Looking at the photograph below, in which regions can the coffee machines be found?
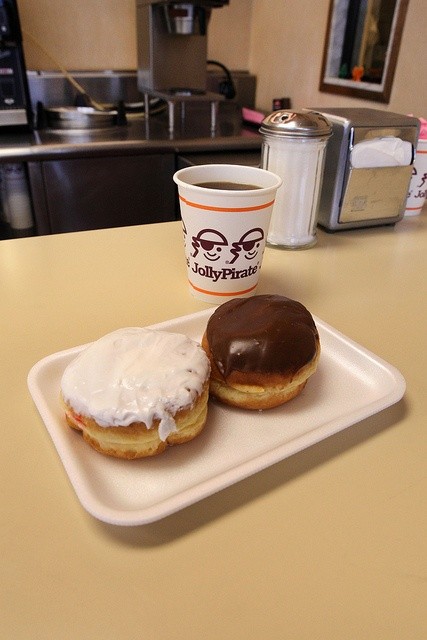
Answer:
[133,2,232,135]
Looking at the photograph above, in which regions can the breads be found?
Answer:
[59,327,210,458]
[202,295,321,411]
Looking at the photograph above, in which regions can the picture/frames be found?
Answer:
[319,1,410,105]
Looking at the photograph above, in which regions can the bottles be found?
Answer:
[3,159,35,229]
[259,109,330,249]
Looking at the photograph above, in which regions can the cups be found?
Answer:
[403,122,427,217]
[173,164,285,305]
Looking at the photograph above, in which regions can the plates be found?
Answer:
[44,103,121,135]
[26,305,407,526]
